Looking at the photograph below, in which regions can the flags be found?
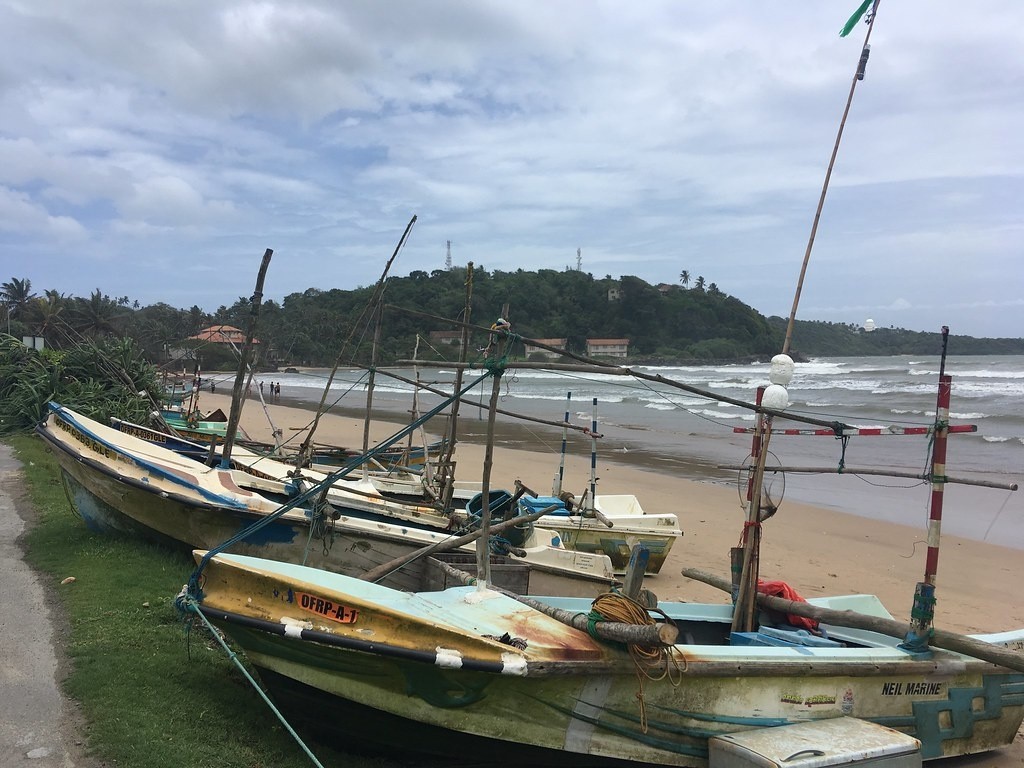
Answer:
[839,0,873,38]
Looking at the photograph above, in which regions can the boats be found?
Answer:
[177,72,1024,767]
[39,214,686,598]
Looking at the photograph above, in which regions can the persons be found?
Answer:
[193,378,215,393]
[275,382,280,398]
[269,381,275,396]
[260,381,264,395]
